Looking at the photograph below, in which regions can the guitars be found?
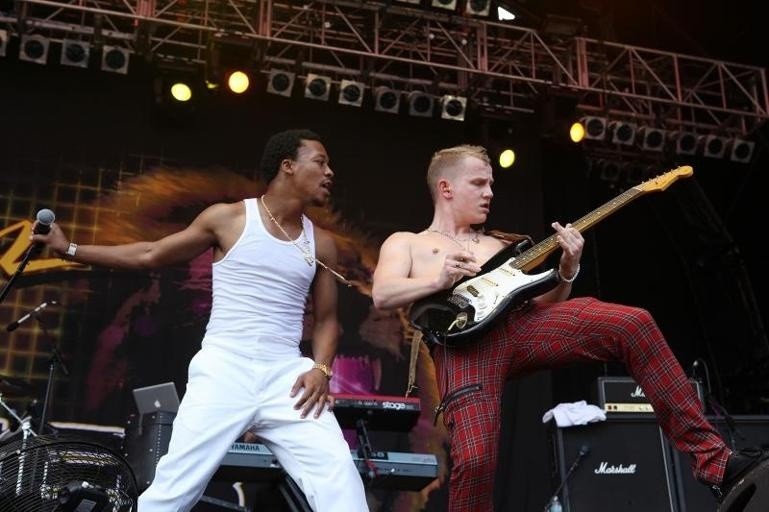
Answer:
[408,165,693,350]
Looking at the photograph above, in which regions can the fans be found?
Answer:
[0,433,139,512]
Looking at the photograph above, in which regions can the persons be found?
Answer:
[372,143,768,512]
[26,127,372,512]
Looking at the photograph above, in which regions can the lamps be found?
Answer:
[161,63,203,108]
[0,28,132,75]
[264,67,474,125]
[461,120,516,170]
[577,113,754,163]
[544,98,588,147]
[213,39,261,99]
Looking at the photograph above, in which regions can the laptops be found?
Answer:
[132,382,180,415]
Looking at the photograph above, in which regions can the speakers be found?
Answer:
[716,457,769,512]
[545,425,682,512]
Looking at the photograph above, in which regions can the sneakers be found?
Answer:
[696,445,768,487]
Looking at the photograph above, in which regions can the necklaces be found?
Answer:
[425,226,479,256]
[257,192,314,267]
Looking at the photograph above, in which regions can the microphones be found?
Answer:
[569,444,589,472]
[7,299,49,332]
[33,207,55,256]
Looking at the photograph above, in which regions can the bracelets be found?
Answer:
[65,242,76,256]
[312,363,332,379]
[558,264,580,283]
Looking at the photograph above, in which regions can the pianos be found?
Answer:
[210,442,437,485]
[329,393,421,433]
